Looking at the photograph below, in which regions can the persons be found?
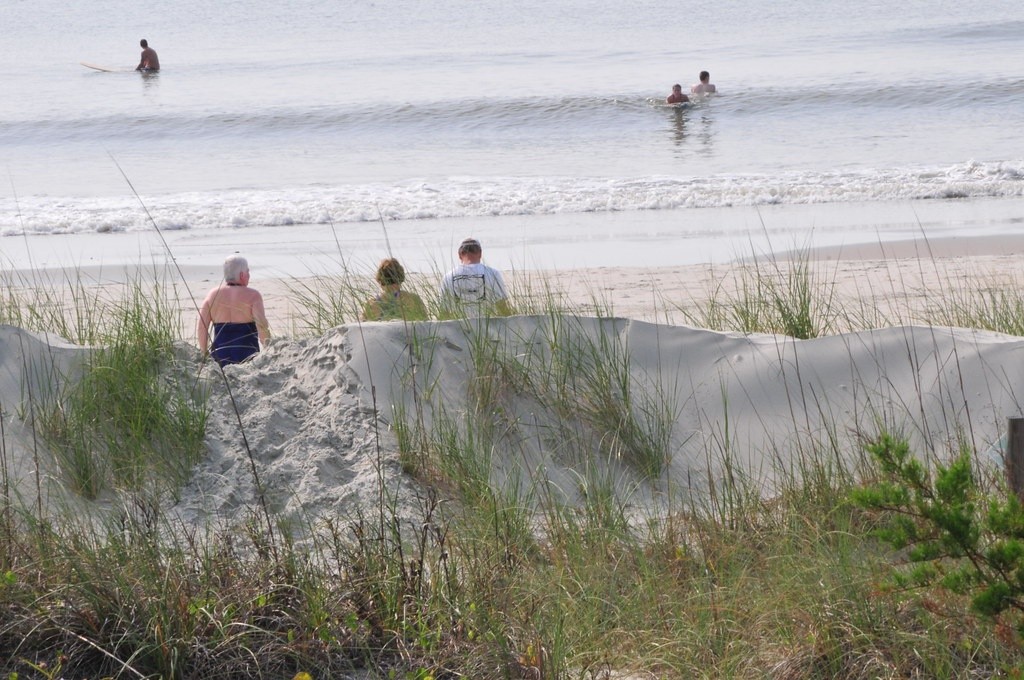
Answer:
[197,254,270,368]
[691,71,715,93]
[440,238,512,320]
[668,84,689,103]
[136,39,160,70]
[362,258,430,321]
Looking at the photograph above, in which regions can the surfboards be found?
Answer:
[79,60,136,72]
